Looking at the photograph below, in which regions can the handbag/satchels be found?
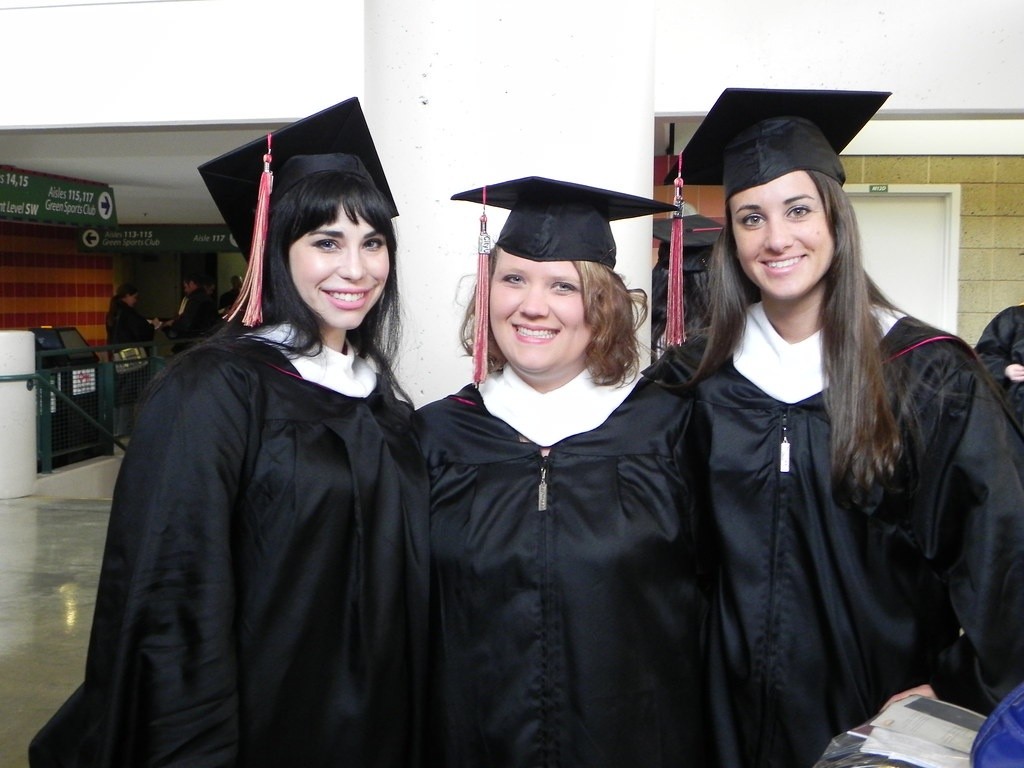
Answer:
[113,346,150,375]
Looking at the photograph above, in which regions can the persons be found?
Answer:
[161,270,243,354]
[412,174,710,768]
[974,303,1024,426]
[105,280,162,436]
[640,86,1024,768]
[652,214,725,362]
[29,95,412,768]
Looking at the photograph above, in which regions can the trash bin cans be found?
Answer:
[29,327,102,474]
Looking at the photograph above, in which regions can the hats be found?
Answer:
[650,214,723,271]
[198,97,397,328]
[452,177,679,382]
[663,88,891,348]
